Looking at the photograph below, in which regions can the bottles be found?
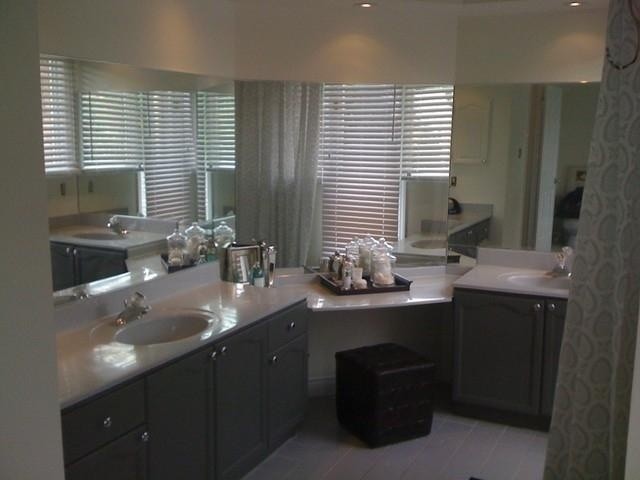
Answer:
[166,220,235,264]
[320,233,397,290]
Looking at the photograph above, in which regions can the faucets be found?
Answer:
[101,216,125,237]
[447,219,462,232]
[447,196,461,214]
[558,246,573,272]
[123,292,146,320]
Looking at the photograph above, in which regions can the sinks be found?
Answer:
[494,265,571,291]
[115,308,214,345]
[412,236,445,250]
[72,229,129,242]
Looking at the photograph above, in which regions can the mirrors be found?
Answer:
[445,81,600,268]
[39,54,282,336]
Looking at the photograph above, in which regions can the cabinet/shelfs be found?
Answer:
[265,298,310,458]
[49,240,168,292]
[450,286,568,432]
[143,321,268,480]
[60,378,149,480]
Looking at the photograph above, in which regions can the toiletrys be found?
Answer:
[165,222,188,264]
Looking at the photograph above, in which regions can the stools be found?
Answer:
[333,341,435,449]
